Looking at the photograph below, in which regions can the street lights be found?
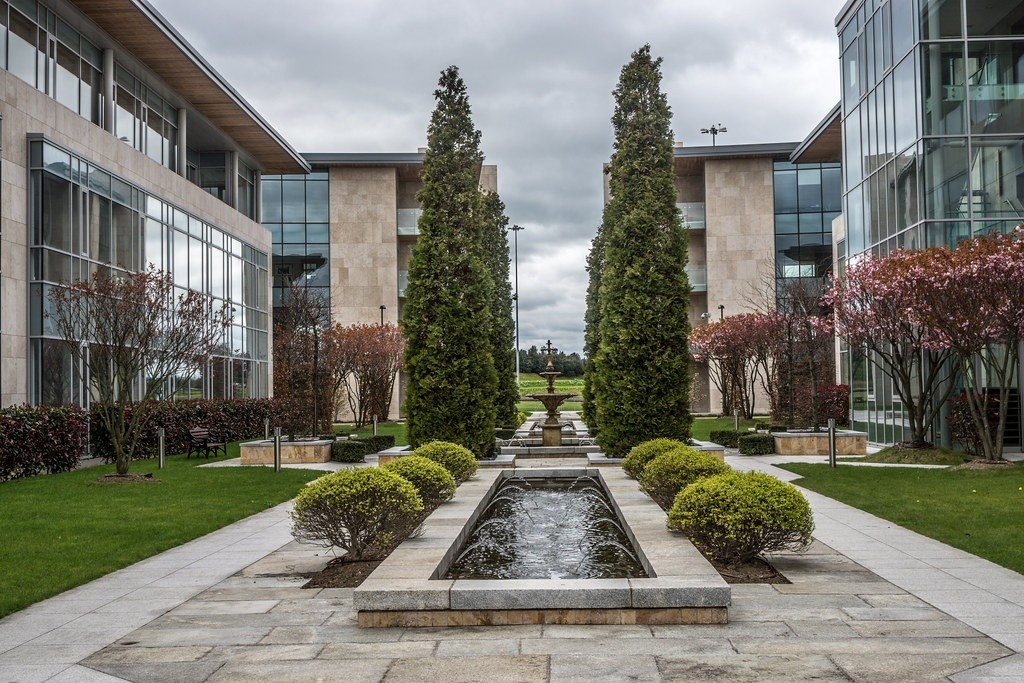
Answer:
[718,304,725,320]
[509,224,525,383]
[379,304,386,327]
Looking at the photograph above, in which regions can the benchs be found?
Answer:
[187,426,227,459]
